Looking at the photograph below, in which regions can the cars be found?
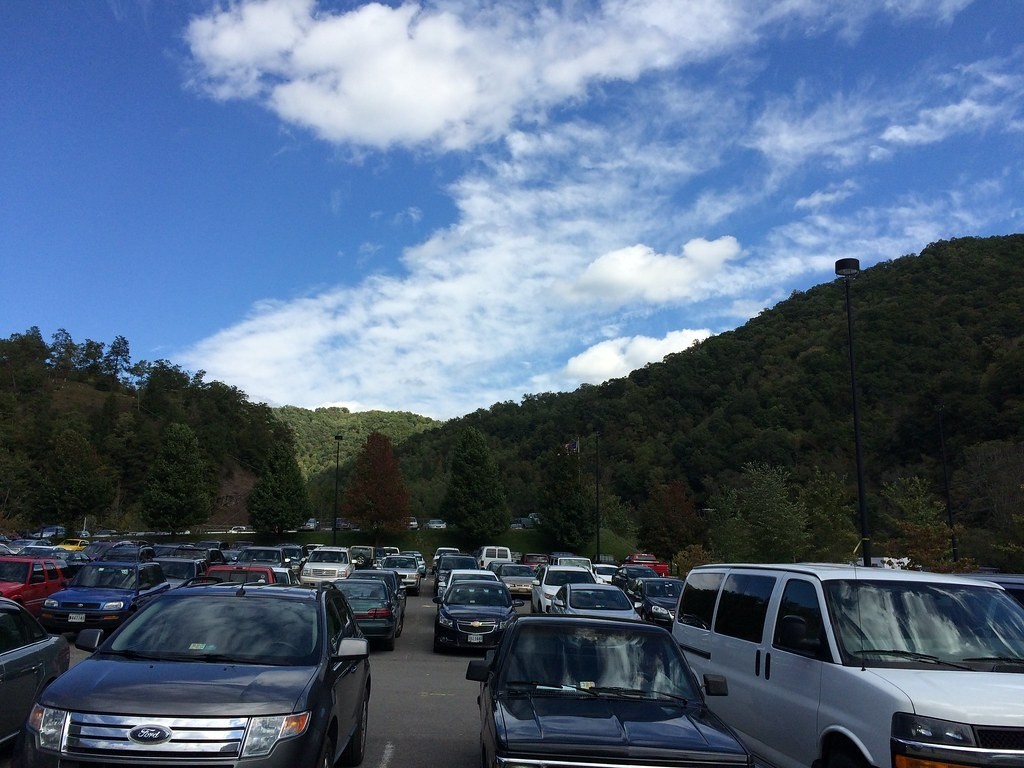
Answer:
[1,524,679,657]
[466,614,753,768]
[0,597,70,760]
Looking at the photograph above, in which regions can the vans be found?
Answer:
[670,561,1023,768]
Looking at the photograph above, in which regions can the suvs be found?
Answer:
[25,584,372,768]
